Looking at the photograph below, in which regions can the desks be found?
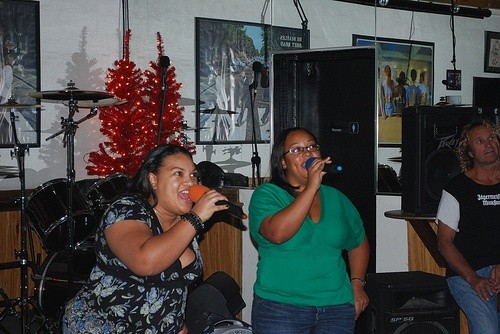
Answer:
[383,208,460,334]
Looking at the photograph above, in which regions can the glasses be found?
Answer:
[284,143,320,156]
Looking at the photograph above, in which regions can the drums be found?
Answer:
[35,241,96,320]
[87,173,140,219]
[24,177,95,253]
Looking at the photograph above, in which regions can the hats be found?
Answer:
[396,71,408,84]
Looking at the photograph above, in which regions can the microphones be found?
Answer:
[253,62,261,97]
[305,158,342,172]
[159,55,170,92]
[188,185,247,220]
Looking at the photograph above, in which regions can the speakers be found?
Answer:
[353,271,461,334]
[402,105,482,216]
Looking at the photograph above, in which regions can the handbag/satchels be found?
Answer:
[378,164,401,193]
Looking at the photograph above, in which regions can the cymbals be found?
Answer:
[0,102,46,109]
[28,90,128,108]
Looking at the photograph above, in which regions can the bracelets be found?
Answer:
[350,278,366,287]
[184,211,204,233]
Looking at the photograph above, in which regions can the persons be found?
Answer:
[417,72,429,105]
[378,65,397,119]
[406,69,421,106]
[435,119,500,334]
[63,143,229,334]
[393,71,406,113]
[248,127,369,334]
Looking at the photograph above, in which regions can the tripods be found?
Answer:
[0,109,55,334]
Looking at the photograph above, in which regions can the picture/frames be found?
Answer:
[484,30,500,73]
[195,17,270,144]
[0,0,41,148]
[352,34,435,147]
[447,69,462,90]
